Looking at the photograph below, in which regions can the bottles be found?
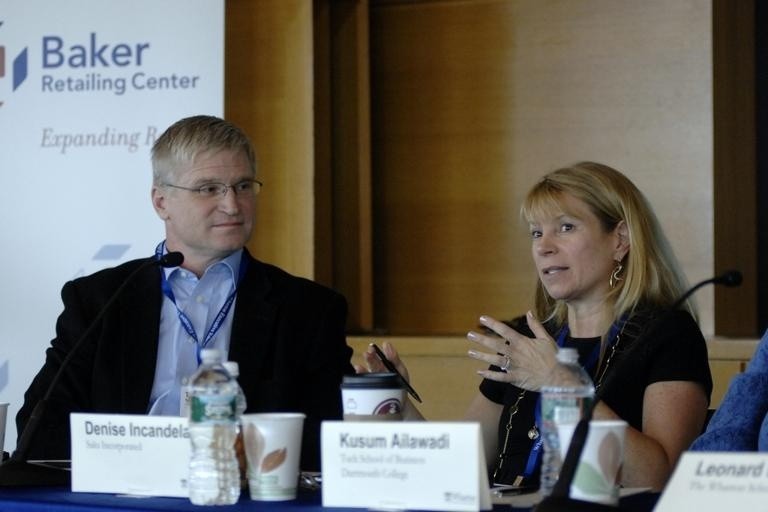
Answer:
[539,344,596,500]
[183,348,248,507]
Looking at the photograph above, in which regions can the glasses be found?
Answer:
[163,180,265,199]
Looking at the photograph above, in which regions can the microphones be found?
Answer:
[532,269,744,512]
[0,251,184,491]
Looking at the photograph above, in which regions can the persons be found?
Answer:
[354,162,713,494]
[11,115,348,471]
[689,328,768,451]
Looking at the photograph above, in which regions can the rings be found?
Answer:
[504,339,510,355]
[501,355,510,371]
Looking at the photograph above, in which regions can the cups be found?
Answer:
[0,402,12,464]
[556,417,628,506]
[340,372,406,417]
[237,413,306,503]
